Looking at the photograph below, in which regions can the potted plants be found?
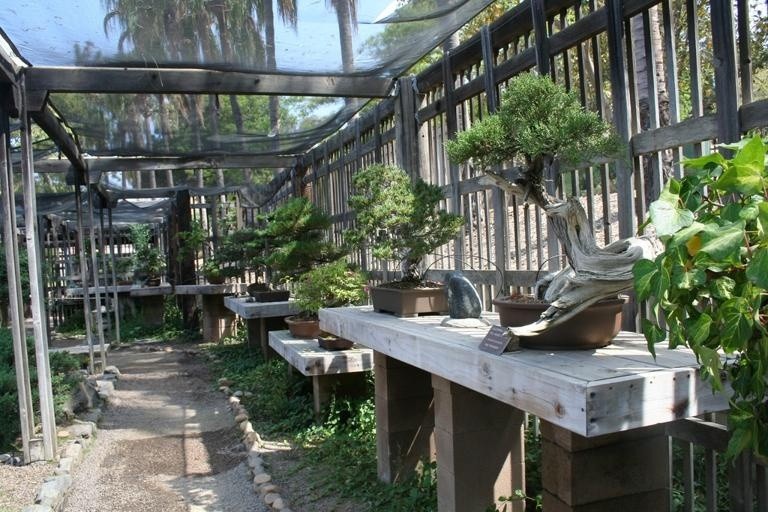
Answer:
[443,71,664,350]
[279,257,369,351]
[223,214,290,303]
[342,162,467,318]
[633,132,768,512]
[173,212,250,284]
[128,224,167,287]
[266,195,353,340]
